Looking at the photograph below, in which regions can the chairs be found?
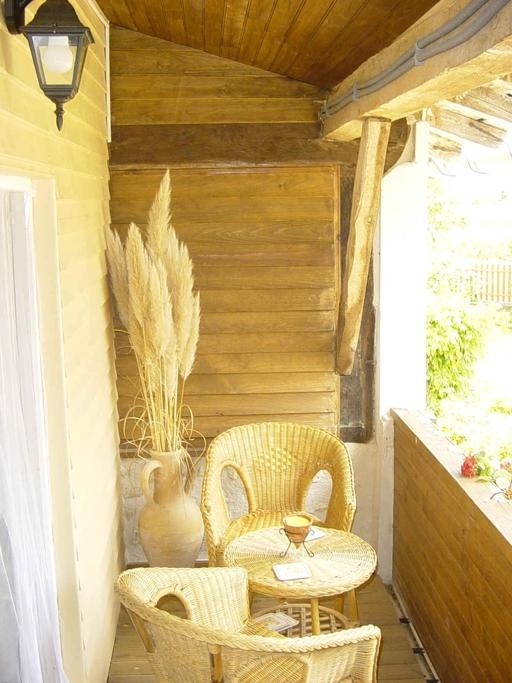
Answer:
[115,566,382,683]
[200,421,357,568]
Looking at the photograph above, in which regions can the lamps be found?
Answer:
[3,0,95,132]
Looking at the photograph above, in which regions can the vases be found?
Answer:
[137,450,206,567]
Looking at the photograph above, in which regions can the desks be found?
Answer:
[222,525,378,636]
